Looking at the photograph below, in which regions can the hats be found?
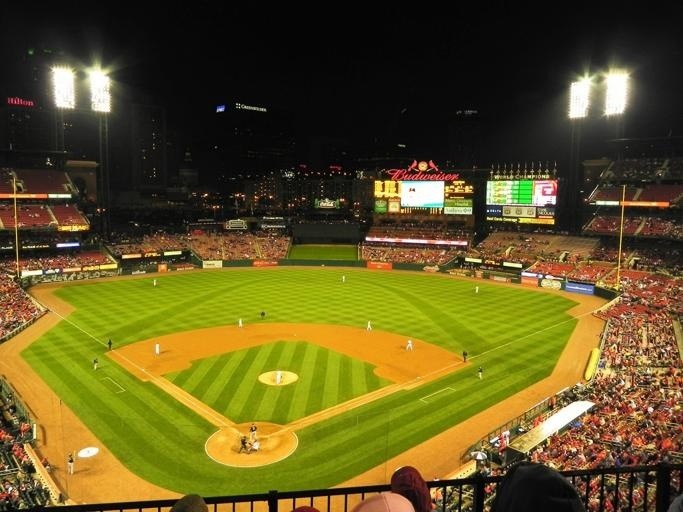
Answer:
[491,463,588,512]
[391,466,432,512]
[349,492,416,512]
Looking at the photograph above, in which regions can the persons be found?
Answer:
[238,317,243,329]
[406,338,413,349]
[106,338,112,352]
[291,506,319,512]
[0,273,77,510]
[92,357,99,371]
[477,366,482,379]
[260,310,265,322]
[275,368,282,384]
[351,465,432,511]
[461,350,468,363]
[356,214,681,296]
[239,424,259,454]
[155,341,159,357]
[365,318,372,331]
[0,202,293,278]
[152,277,157,287]
[426,412,683,511]
[341,273,345,283]
[549,295,682,412]
